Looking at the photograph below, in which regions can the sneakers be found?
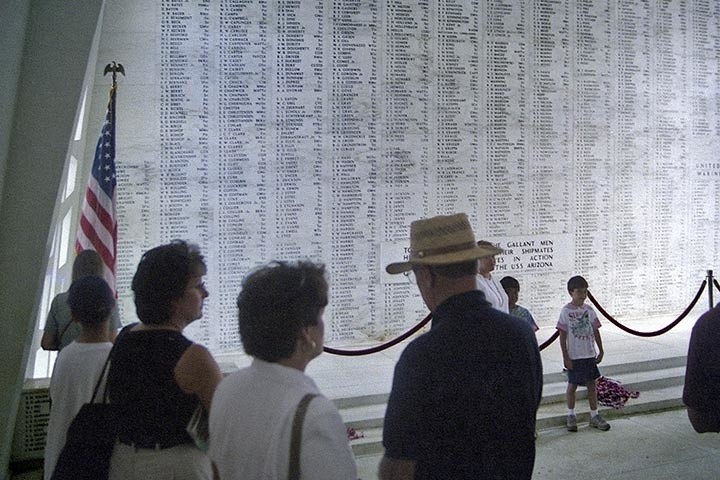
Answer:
[567,415,578,432]
[589,415,610,430]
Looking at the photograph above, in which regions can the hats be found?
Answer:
[387,213,503,275]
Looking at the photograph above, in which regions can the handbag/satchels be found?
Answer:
[50,322,139,480]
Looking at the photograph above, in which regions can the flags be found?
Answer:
[75,82,118,300]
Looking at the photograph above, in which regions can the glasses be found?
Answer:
[404,269,422,284]
[186,282,206,291]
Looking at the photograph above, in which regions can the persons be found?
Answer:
[682,303,720,433]
[475,240,509,314]
[378,213,544,480]
[501,276,539,332]
[556,275,610,432]
[40,250,121,350]
[45,276,114,480]
[206,260,357,480]
[109,242,219,480]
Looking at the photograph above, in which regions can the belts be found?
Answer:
[120,439,192,449]
